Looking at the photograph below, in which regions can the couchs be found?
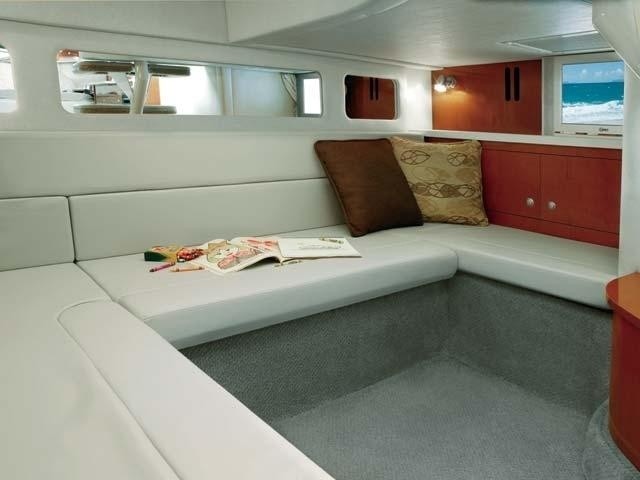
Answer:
[375,130,619,416]
[1,132,333,480]
[68,134,454,421]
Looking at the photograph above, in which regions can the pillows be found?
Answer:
[313,139,425,238]
[391,138,488,226]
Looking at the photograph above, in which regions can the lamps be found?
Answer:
[435,74,458,92]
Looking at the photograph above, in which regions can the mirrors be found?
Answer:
[343,71,400,122]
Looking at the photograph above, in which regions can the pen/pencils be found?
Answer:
[148,261,205,272]
[274,260,302,267]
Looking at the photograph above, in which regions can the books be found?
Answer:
[182,235,363,276]
